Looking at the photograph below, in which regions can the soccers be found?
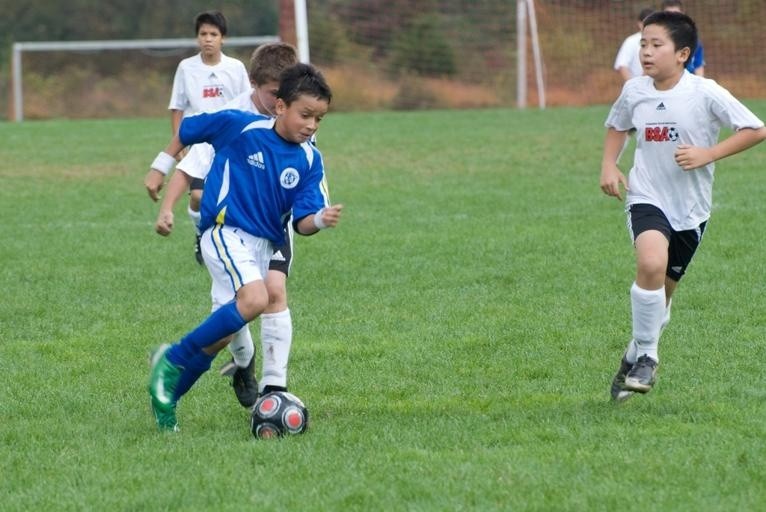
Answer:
[251,391,308,440]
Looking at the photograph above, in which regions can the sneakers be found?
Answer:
[151,397,180,435]
[148,341,186,410]
[625,353,658,392]
[222,350,260,407]
[611,351,636,402]
[194,234,204,267]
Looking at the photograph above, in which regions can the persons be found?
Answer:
[659,0,706,76]
[615,7,654,82]
[167,12,254,267]
[597,10,765,408]
[152,44,315,413]
[141,61,345,437]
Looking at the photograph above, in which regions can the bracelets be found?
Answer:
[313,206,329,232]
[149,150,177,176]
